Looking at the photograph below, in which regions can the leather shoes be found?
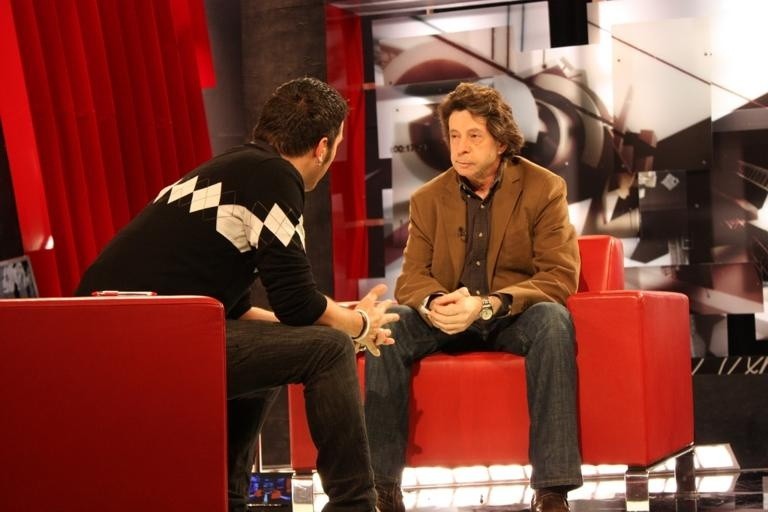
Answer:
[531,487,571,512]
[373,481,406,512]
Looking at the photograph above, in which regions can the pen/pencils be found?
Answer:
[91,290,157,296]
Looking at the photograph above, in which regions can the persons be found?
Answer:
[363,77,590,511]
[71,76,402,512]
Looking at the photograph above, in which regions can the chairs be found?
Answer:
[286,235,697,511]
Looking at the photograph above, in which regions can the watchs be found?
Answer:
[477,293,495,323]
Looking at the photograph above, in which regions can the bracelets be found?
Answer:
[355,306,371,345]
